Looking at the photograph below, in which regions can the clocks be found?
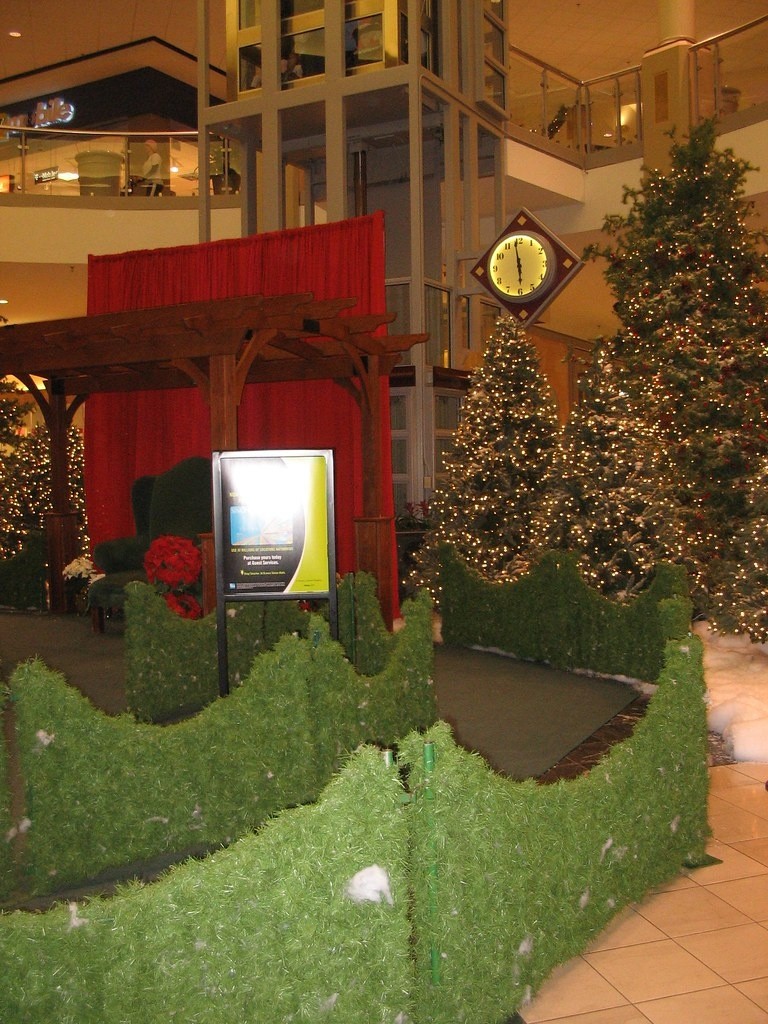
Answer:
[468,206,585,328]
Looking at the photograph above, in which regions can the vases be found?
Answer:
[76,599,86,612]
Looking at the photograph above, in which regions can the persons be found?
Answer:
[142,140,163,196]
[281,48,303,81]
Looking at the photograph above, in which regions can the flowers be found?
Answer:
[61,557,98,598]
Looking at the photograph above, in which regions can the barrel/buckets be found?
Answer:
[75,150,123,197]
